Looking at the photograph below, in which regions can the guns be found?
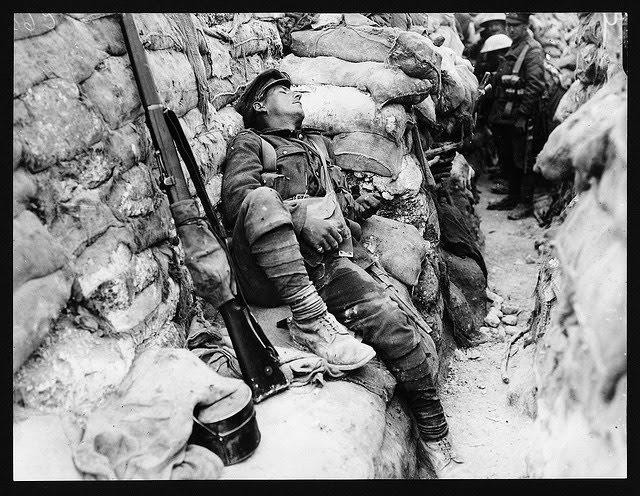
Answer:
[118,11,289,403]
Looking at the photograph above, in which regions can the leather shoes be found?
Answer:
[507,203,534,219]
[491,182,512,194]
[416,436,491,481]
[290,314,377,370]
[489,196,518,209]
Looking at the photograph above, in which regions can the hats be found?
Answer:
[505,11,530,25]
[233,69,291,111]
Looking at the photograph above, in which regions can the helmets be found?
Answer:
[479,14,506,26]
[481,34,513,53]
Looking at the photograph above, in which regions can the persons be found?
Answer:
[464,11,547,222]
[219,68,469,479]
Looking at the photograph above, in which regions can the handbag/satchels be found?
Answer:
[282,139,351,240]
